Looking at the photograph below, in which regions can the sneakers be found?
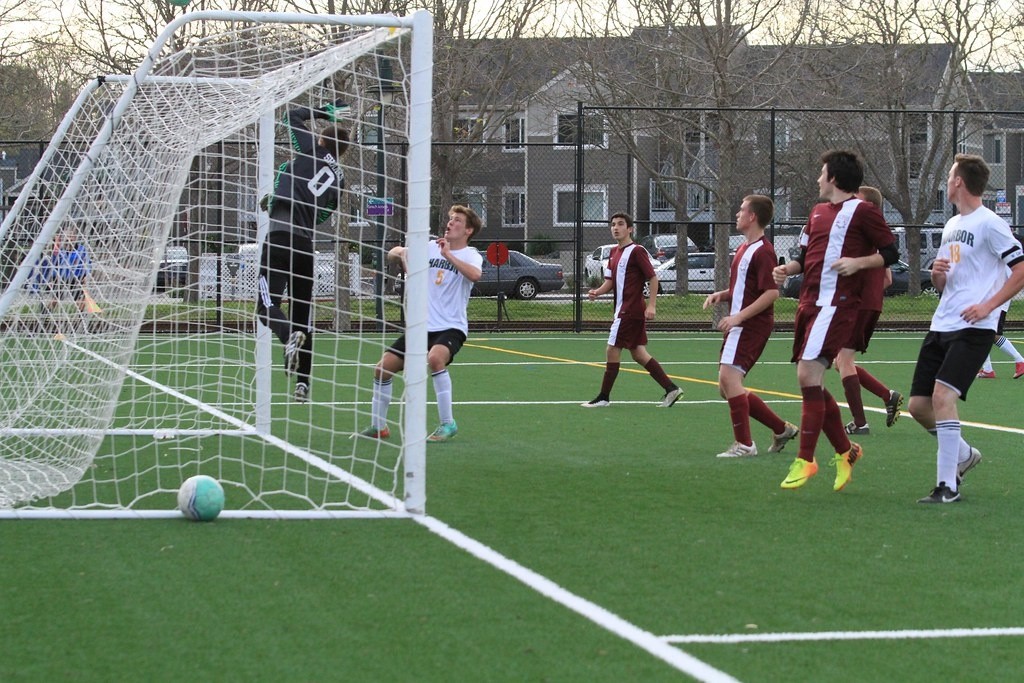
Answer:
[284,331,306,377]
[780,456,818,489]
[580,395,610,407]
[360,423,390,438]
[976,369,995,378]
[656,387,684,408]
[956,446,983,486]
[828,441,863,491]
[844,421,869,434]
[1012,360,1024,378]
[916,481,961,502]
[293,382,309,402]
[884,390,904,427]
[768,421,799,452]
[427,420,457,443]
[716,440,757,457]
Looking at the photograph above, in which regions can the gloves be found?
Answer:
[321,97,351,122]
[259,194,268,212]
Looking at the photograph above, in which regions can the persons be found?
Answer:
[975,264,1024,379]
[256,97,353,401]
[833,186,903,435]
[580,212,684,408]
[907,152,1024,504]
[703,195,799,457]
[772,148,904,492]
[358,204,484,444]
[28,221,90,317]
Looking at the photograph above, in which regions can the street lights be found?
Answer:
[364,49,403,329]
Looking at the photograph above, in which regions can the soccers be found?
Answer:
[178,474,226,522]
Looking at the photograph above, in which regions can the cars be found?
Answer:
[153,245,188,286]
[782,260,941,299]
[638,233,699,264]
[392,250,564,299]
[889,228,943,270]
[585,244,661,283]
[642,252,736,299]
